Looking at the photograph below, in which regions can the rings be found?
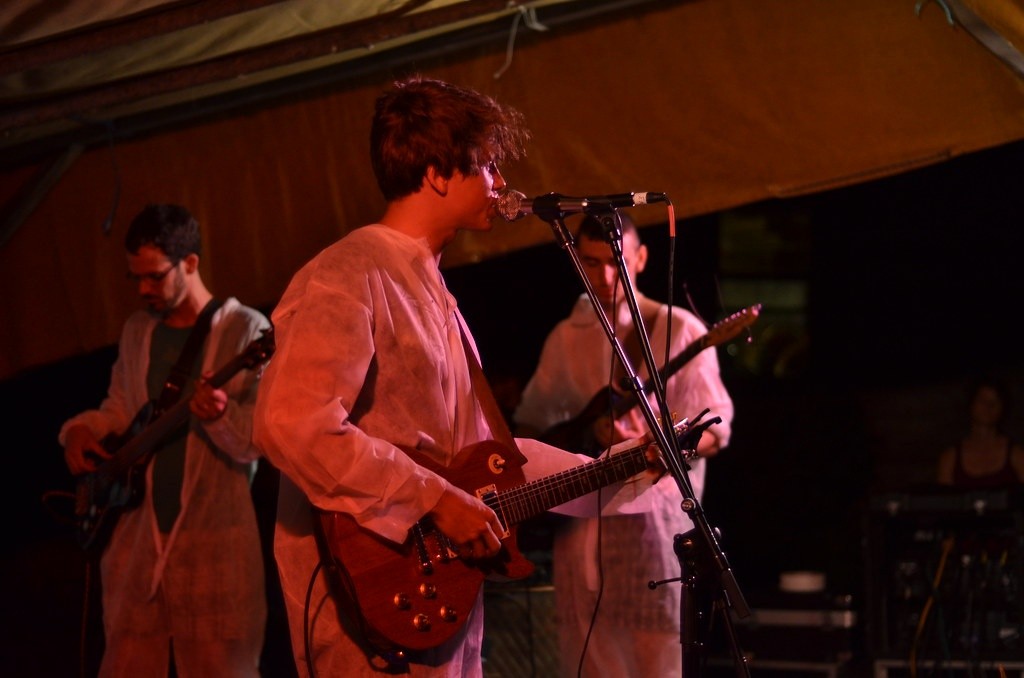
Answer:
[200,402,209,410]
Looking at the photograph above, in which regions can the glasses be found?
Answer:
[126,258,180,282]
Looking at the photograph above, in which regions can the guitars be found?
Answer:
[316,406,723,666]
[538,301,764,462]
[32,327,275,570]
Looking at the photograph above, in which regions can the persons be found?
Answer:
[252,69,668,678]
[934,378,1024,563]
[511,208,735,677]
[58,199,276,678]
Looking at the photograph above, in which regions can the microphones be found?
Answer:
[495,189,667,223]
